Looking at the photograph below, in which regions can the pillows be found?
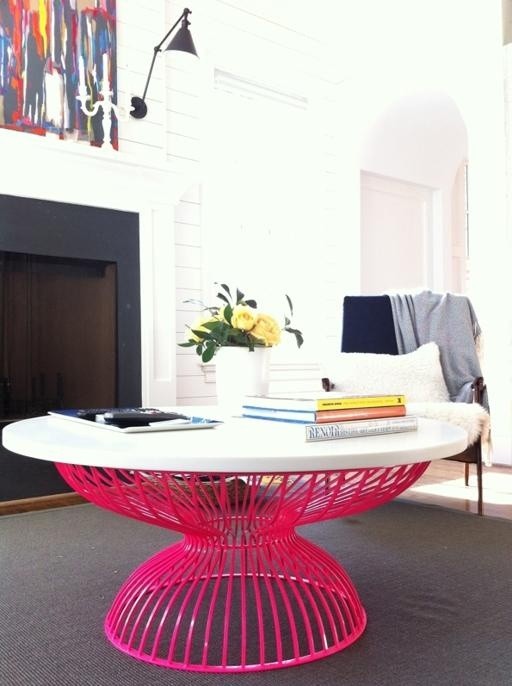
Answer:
[337,341,451,404]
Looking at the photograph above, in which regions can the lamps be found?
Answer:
[130,6,202,121]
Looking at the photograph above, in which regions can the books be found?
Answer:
[230,391,418,445]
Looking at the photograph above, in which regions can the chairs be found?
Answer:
[340,294,493,517]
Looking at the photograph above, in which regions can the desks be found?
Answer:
[2,405,469,676]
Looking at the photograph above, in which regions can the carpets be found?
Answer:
[0,480,511,686]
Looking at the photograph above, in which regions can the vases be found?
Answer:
[214,346,256,418]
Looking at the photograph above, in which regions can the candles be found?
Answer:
[78,52,109,89]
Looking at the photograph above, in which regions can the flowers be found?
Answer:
[178,277,304,365]
